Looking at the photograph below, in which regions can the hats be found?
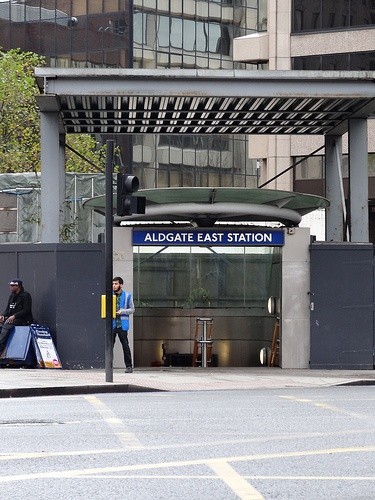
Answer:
[9,279,23,286]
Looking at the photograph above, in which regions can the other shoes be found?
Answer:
[125,367,133,373]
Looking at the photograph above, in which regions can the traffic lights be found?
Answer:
[117,172,146,217]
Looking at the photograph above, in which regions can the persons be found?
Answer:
[112,277,135,373]
[0,280,33,352]
